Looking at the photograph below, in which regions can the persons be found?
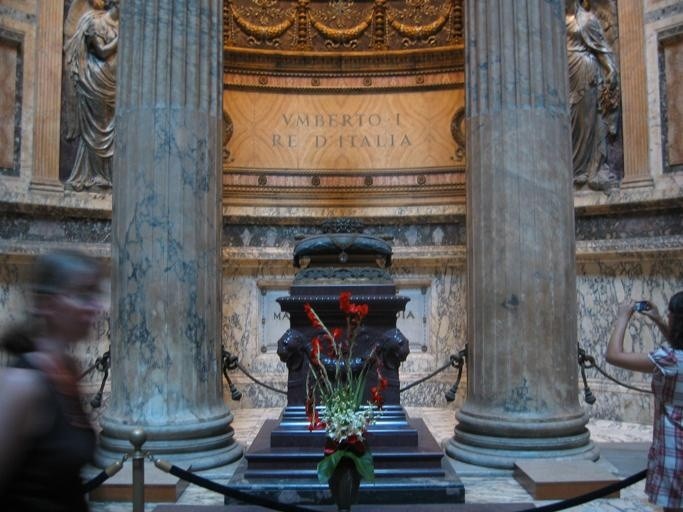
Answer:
[65,3,119,192]
[0,250,111,512]
[566,0,619,187]
[605,289,683,511]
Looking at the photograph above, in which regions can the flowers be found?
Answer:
[303,291,388,487]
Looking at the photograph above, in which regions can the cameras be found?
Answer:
[633,300,649,312]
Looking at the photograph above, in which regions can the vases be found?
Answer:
[328,456,360,512]
